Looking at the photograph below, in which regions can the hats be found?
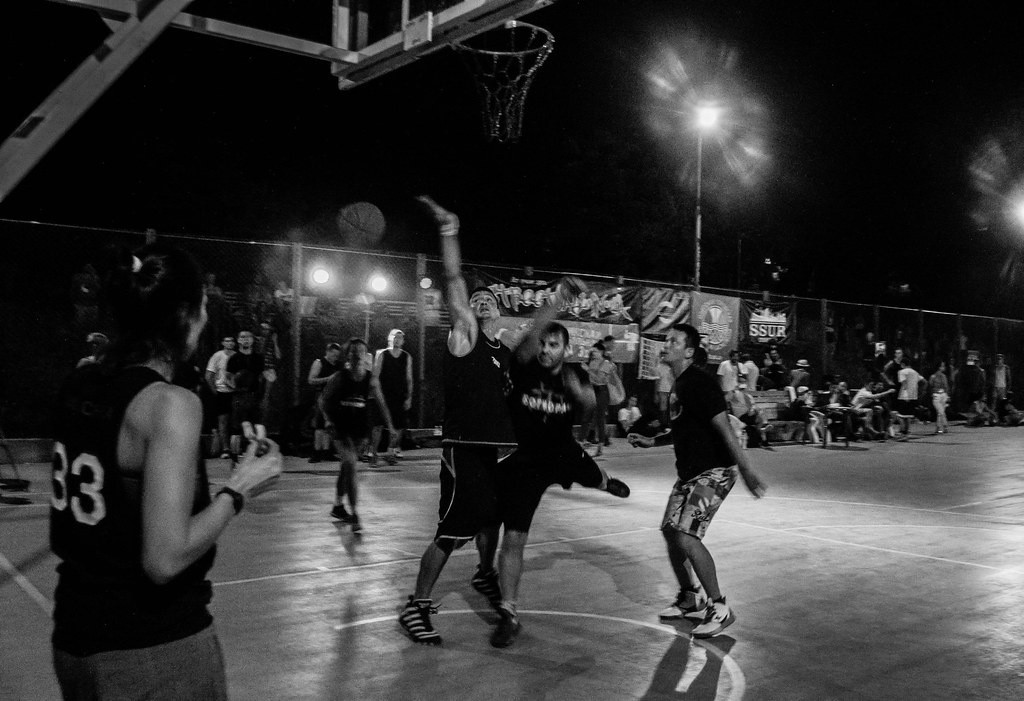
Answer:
[796,359,810,367]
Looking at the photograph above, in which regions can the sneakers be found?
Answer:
[605,478,630,498]
[399,595,442,647]
[661,586,706,620]
[690,597,735,639]
[491,617,521,648]
[471,563,502,610]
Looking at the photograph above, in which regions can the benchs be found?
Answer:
[749,388,865,442]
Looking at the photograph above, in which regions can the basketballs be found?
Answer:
[339,202,386,248]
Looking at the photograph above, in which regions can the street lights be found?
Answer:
[689,99,721,294]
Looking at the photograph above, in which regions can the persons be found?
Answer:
[375,329,412,466]
[717,348,1013,445]
[74,328,111,367]
[617,394,669,438]
[318,337,398,532]
[580,335,624,450]
[308,344,342,463]
[993,353,1011,427]
[51,241,284,700]
[627,324,764,638]
[225,330,268,455]
[488,276,629,647]
[204,338,236,460]
[898,359,927,435]
[929,359,951,433]
[399,199,524,648]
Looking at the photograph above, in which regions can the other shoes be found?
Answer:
[352,514,361,532]
[220,449,230,458]
[331,505,352,521]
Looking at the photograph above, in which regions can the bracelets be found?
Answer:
[216,487,244,516]
[441,224,459,238]
